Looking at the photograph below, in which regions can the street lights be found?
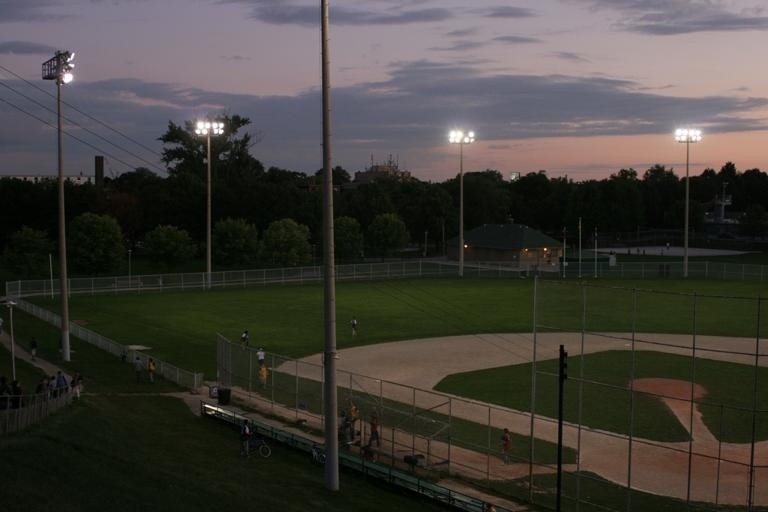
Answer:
[2,300,17,382]
[41,50,75,364]
[675,127,702,279]
[447,129,476,280]
[194,120,225,291]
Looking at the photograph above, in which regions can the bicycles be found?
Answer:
[312,446,327,466]
[248,439,271,458]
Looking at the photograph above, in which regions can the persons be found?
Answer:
[346,401,360,440]
[257,364,270,391]
[368,406,381,447]
[239,418,250,458]
[350,316,357,336]
[241,330,249,350]
[133,357,145,385]
[257,345,265,365]
[146,358,157,383]
[500,428,512,464]
[29,337,38,361]
[1,369,86,409]
